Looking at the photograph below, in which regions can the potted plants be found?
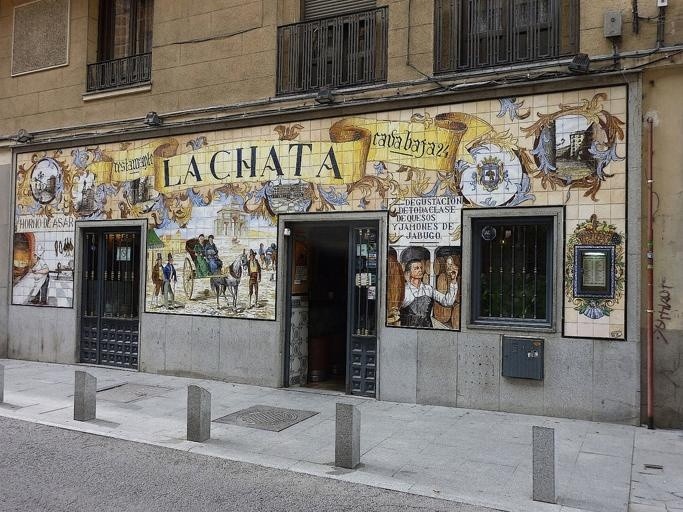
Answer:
[308,309,334,382]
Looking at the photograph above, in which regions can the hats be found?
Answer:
[249,249,257,256]
[33,244,45,259]
[156,253,162,261]
[168,252,173,261]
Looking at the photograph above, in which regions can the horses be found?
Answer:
[209,248,250,309]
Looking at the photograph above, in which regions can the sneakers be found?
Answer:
[31,298,46,305]
[154,303,182,312]
[247,304,261,309]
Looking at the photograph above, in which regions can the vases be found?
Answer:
[329,335,346,376]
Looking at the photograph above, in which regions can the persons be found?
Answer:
[398,257,458,328]
[29,252,50,305]
[150,233,278,310]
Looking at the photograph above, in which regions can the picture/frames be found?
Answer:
[11,0,70,78]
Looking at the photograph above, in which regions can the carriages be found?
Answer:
[182,238,248,309]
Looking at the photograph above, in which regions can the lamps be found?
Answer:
[284,222,291,237]
[567,53,590,74]
[145,112,162,127]
[15,129,35,142]
[315,85,334,104]
